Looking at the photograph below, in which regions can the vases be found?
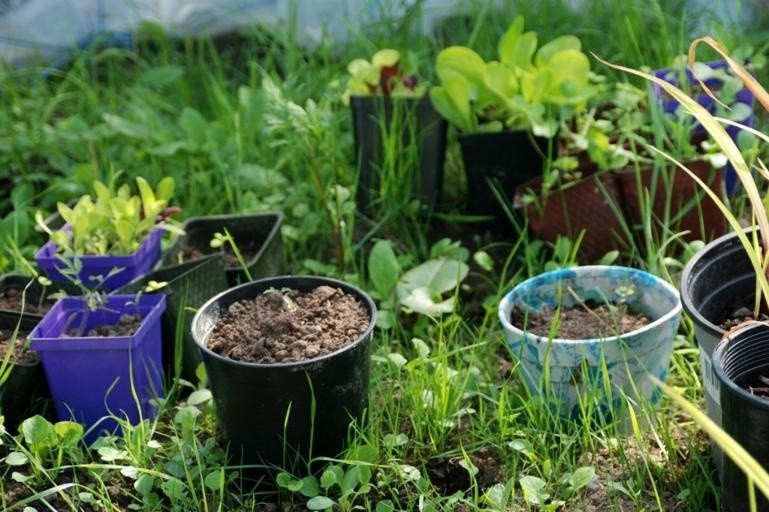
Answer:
[187,273,380,463]
[711,323,769,511]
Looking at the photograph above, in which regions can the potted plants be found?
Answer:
[35,172,178,297]
[0,273,58,312]
[596,34,769,426]
[0,312,53,425]
[341,17,764,266]
[30,295,173,442]
[182,211,292,278]
[112,255,227,392]
[496,264,684,426]
